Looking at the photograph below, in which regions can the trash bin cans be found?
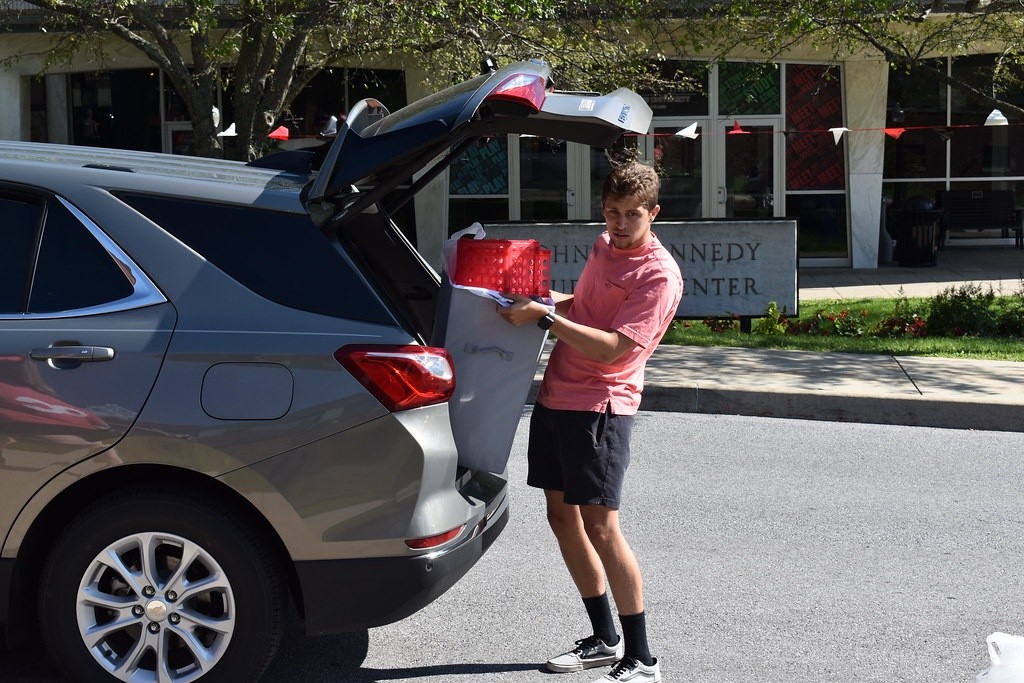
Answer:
[898,195,953,269]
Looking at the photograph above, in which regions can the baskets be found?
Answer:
[454,236,552,299]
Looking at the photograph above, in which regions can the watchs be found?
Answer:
[537,311,555,331]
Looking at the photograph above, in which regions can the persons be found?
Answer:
[496,160,683,683]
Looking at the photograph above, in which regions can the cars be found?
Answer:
[487,149,701,220]
[727,159,897,226]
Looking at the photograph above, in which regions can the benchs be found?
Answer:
[935,188,1024,252]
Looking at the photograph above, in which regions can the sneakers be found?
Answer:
[588,656,663,683]
[547,634,623,672]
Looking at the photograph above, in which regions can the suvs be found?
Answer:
[0,61,657,683]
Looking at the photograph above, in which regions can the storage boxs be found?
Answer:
[429,238,556,474]
